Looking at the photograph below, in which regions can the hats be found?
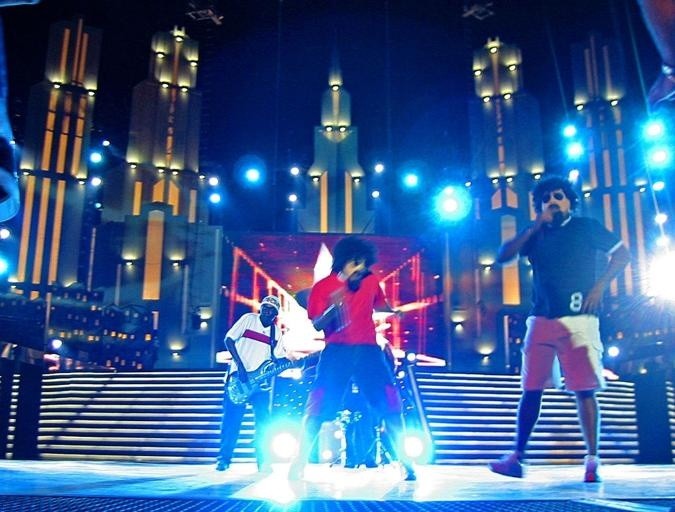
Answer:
[260,295,280,316]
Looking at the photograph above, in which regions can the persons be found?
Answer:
[286,237,421,482]
[210,294,282,472]
[487,174,633,485]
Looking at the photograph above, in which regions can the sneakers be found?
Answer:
[287,462,305,481]
[215,460,230,472]
[488,453,524,479]
[258,464,276,473]
[400,463,417,481]
[583,457,603,483]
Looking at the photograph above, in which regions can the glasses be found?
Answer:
[542,192,564,204]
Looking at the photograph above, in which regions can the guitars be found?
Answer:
[225,358,305,406]
